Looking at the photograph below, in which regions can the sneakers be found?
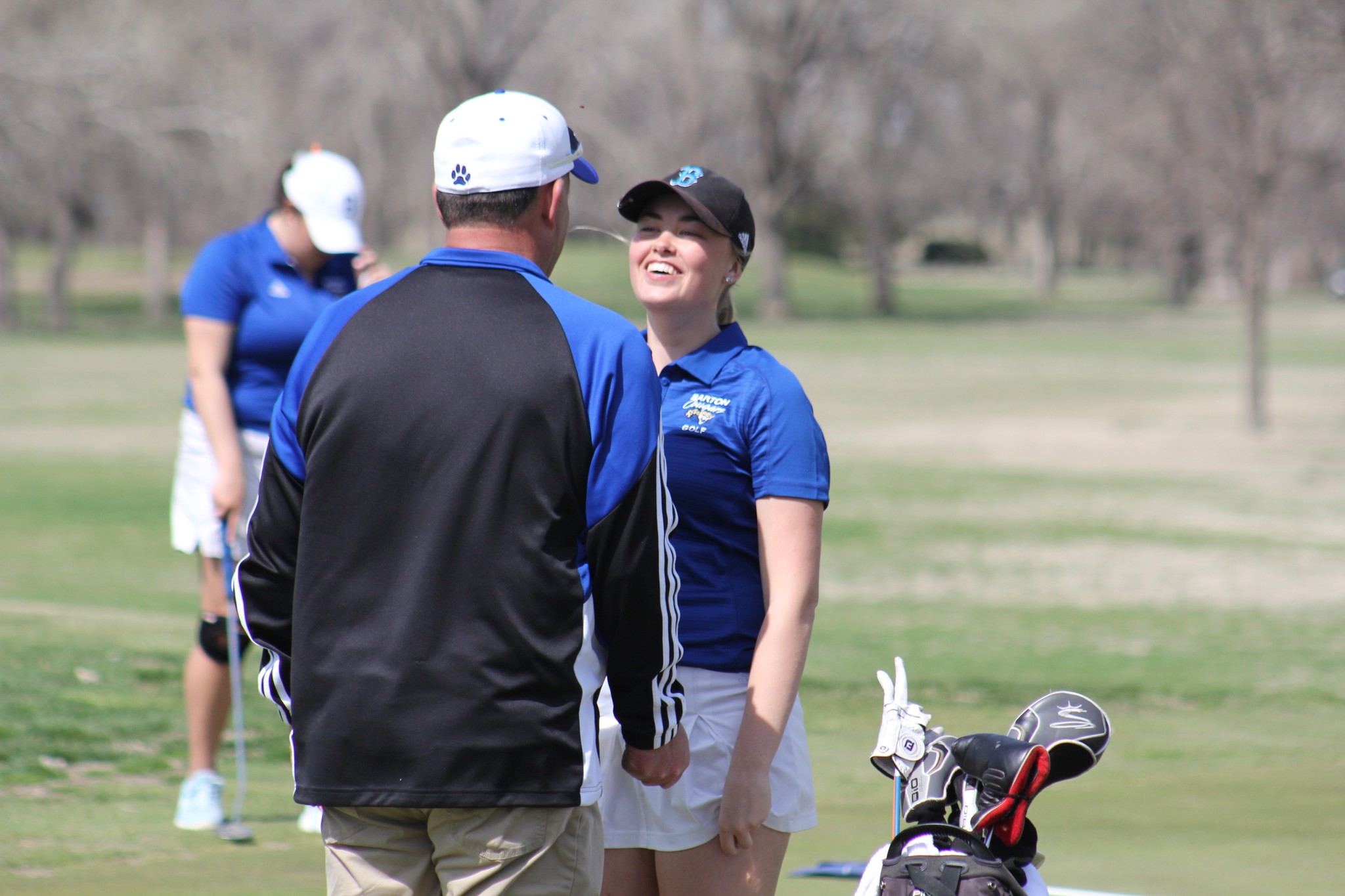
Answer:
[173,770,229,830]
[297,805,323,833]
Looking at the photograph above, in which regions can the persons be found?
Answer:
[567,164,832,896]
[228,88,693,895]
[167,146,387,838]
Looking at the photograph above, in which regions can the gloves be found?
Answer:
[870,657,944,782]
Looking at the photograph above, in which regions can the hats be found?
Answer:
[282,151,365,254]
[618,165,755,270]
[433,90,599,195]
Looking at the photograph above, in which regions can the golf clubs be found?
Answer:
[216,516,256,844]
